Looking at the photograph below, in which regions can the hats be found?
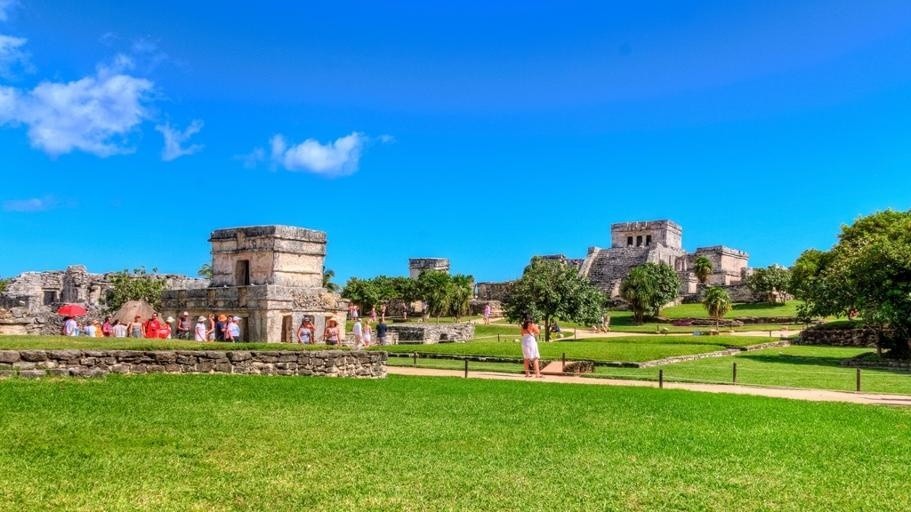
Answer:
[135,311,242,322]
[326,318,339,325]
[304,317,310,322]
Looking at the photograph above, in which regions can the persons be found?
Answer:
[402,302,407,319]
[349,303,387,347]
[482,304,490,324]
[549,321,561,332]
[595,314,610,332]
[61,311,242,342]
[297,317,316,344]
[324,317,341,344]
[421,300,427,321]
[520,313,541,379]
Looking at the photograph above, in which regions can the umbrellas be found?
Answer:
[58,305,88,318]
